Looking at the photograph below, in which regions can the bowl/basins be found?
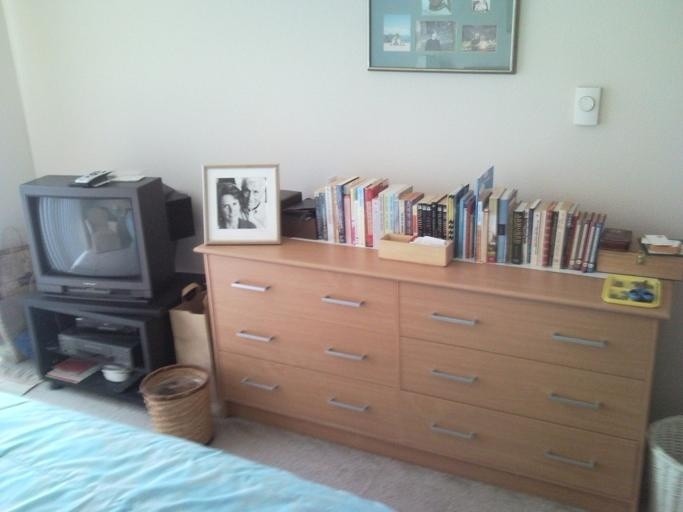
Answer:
[101,364,132,383]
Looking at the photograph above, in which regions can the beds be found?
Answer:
[0,392,395,512]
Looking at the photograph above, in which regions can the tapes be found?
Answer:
[101,364,132,382]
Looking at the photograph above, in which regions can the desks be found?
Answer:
[21,273,205,403]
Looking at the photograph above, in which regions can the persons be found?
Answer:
[423,29,440,50]
[216,182,255,230]
[238,178,266,230]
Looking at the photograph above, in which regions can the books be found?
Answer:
[641,236,681,255]
[312,172,607,273]
[600,226,633,251]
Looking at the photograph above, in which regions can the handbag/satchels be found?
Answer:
[169,283,226,423]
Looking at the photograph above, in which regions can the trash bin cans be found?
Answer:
[646,414,683,512]
[137,363,213,445]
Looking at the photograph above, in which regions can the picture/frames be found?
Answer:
[368,0,520,75]
[202,162,282,246]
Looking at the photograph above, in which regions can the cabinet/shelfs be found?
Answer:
[193,235,673,512]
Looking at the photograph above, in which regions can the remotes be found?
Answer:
[75,168,113,184]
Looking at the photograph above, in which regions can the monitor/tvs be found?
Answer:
[18,175,195,305]
